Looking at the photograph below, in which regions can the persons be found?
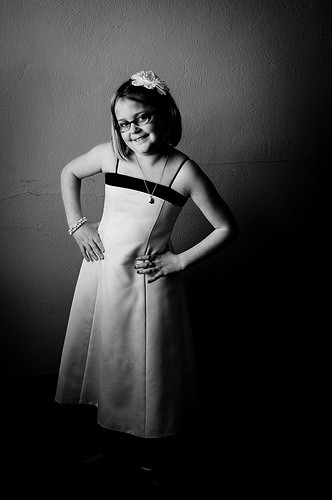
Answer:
[54,70,239,500]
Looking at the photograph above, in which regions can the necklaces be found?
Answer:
[133,149,171,203]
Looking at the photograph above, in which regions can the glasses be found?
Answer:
[113,111,153,132]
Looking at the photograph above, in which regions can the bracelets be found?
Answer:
[68,216,87,235]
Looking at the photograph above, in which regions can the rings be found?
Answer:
[148,261,152,267]
